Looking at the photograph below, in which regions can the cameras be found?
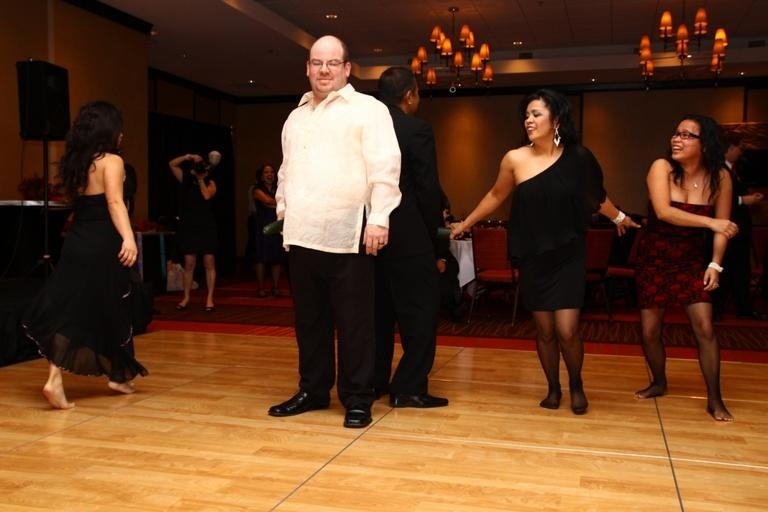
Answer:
[192,160,206,178]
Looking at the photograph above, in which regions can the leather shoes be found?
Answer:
[343,404,372,429]
[371,385,390,400]
[205,303,215,312]
[175,301,190,311]
[267,389,332,416]
[389,389,449,408]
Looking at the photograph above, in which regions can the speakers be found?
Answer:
[17,58,71,142]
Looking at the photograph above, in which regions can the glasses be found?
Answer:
[670,130,700,142]
[310,59,341,72]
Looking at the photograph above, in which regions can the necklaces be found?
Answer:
[679,168,705,188]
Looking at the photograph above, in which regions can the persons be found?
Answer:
[376,67,450,408]
[713,132,767,320]
[446,89,642,414]
[629,115,741,421]
[422,205,464,323]
[243,164,292,297]
[169,152,221,313]
[19,100,139,410]
[117,147,136,217]
[268,35,404,427]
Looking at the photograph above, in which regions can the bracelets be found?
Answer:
[707,261,724,272]
[198,179,203,183]
[612,210,626,225]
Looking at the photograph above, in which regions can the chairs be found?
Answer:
[587,229,636,321]
[467,221,521,328]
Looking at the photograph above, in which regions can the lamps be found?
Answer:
[410,7,494,88]
[639,0,728,92]
